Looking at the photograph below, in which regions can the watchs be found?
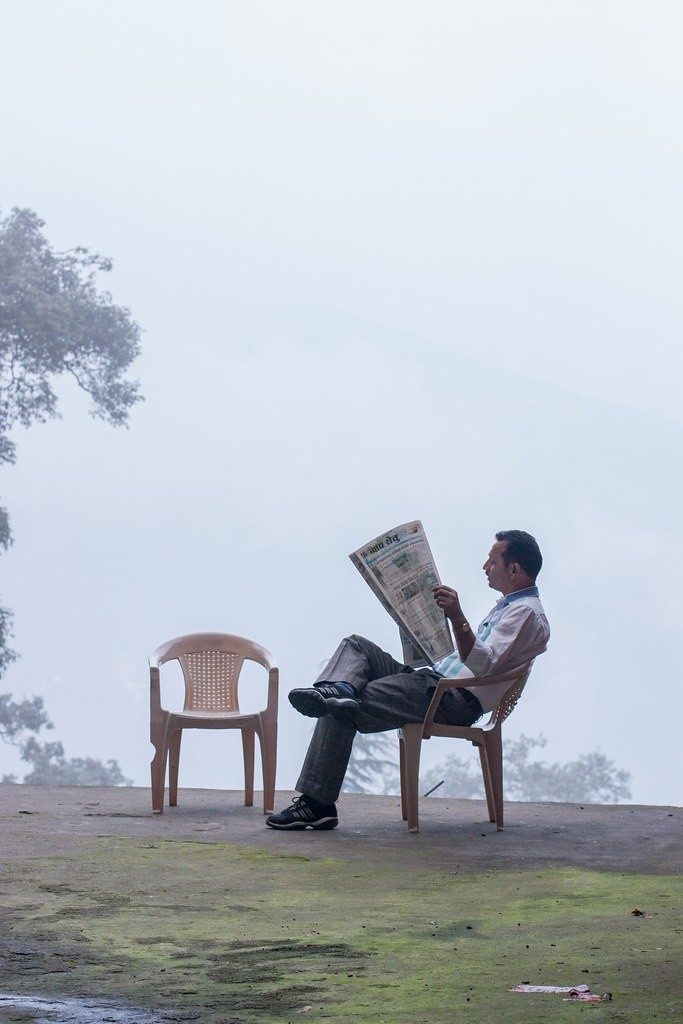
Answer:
[453,623,471,634]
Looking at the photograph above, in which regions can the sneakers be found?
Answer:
[266,794,338,830]
[288,683,359,717]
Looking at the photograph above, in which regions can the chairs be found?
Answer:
[148,633,280,814]
[396,658,535,831]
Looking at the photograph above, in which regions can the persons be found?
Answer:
[266,530,550,830]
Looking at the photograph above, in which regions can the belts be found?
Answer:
[455,687,481,719]
